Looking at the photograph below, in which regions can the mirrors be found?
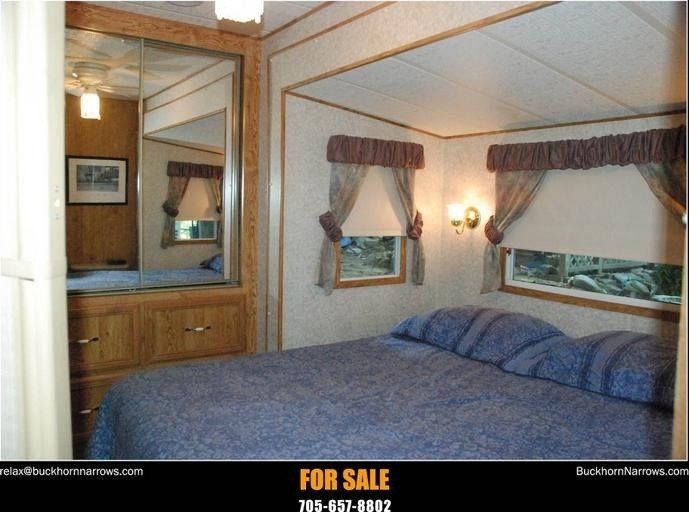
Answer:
[64,3,265,298]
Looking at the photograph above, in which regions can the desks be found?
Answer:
[69,258,132,274]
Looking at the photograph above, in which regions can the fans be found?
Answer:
[63,62,141,98]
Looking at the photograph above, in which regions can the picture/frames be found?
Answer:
[65,154,129,206]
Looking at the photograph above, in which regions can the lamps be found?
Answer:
[78,92,101,118]
[447,198,479,238]
[212,2,267,26]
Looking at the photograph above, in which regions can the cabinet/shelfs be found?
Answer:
[62,292,261,461]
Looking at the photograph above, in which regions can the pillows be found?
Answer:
[202,252,223,274]
[392,303,571,370]
[514,329,675,411]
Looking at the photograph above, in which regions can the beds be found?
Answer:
[68,251,223,292]
[90,301,681,464]
